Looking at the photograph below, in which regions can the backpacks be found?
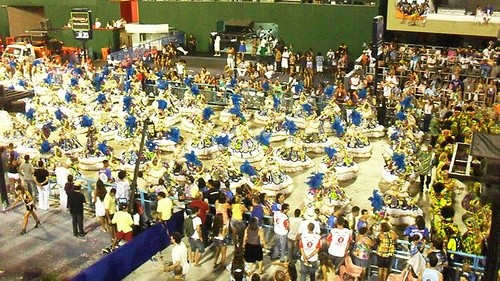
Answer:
[184,215,197,237]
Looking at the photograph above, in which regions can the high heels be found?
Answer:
[34,221,41,228]
[20,230,26,235]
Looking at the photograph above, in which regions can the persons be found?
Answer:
[0,0,499,281]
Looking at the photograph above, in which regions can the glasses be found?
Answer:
[284,208,290,210]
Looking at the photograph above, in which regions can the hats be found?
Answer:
[73,178,82,186]
